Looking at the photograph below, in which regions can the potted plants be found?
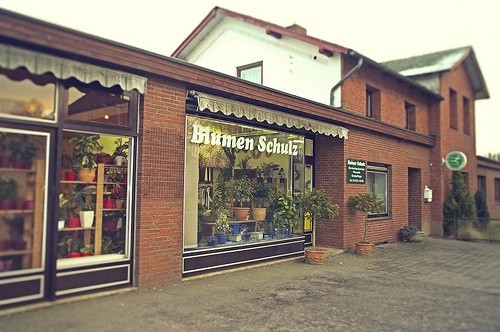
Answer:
[399,223,426,243]
[57,134,129,259]
[0,131,36,277]
[198,149,298,246]
[348,192,385,255]
[298,187,340,265]
